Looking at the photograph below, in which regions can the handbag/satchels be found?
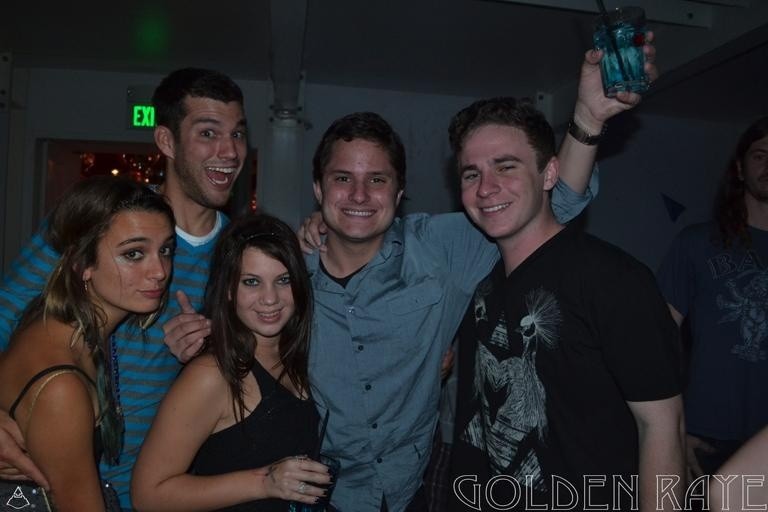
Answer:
[0,370,121,510]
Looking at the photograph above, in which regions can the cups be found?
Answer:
[290,457,340,512]
[595,27,648,97]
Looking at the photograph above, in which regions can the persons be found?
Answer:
[0,32,768,512]
